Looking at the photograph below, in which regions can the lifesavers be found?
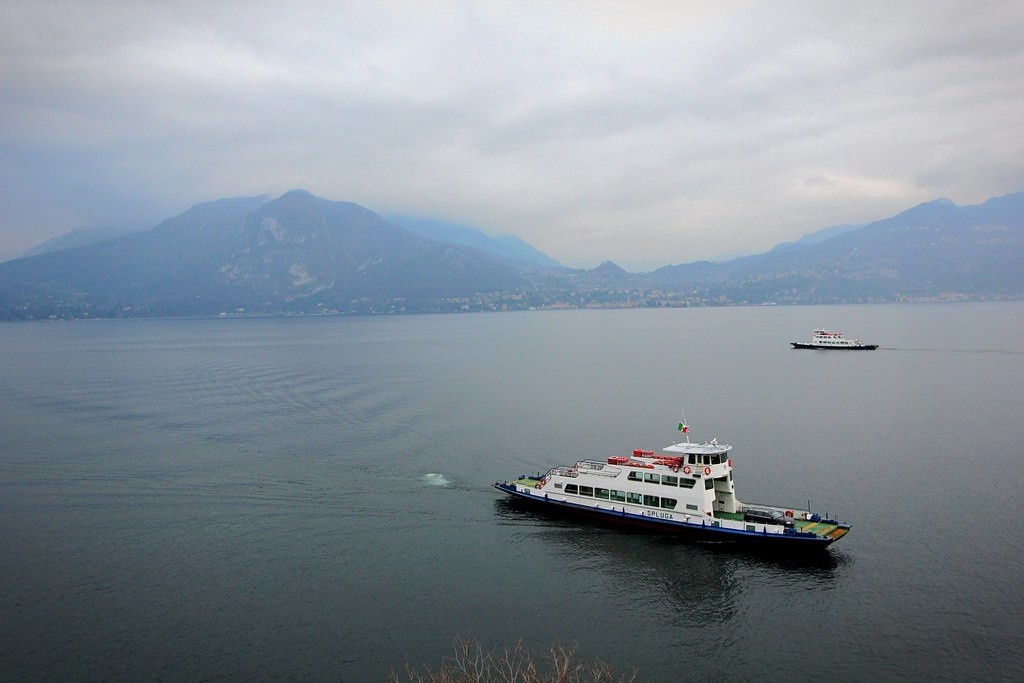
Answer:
[535,483,541,489]
[540,478,546,485]
[683,466,691,473]
[672,465,679,473]
[785,511,793,518]
[705,467,711,475]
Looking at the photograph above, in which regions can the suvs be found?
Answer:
[744,509,796,527]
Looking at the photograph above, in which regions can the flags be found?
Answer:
[677,421,688,433]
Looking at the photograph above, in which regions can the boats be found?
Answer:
[493,408,854,555]
[790,326,879,350]
